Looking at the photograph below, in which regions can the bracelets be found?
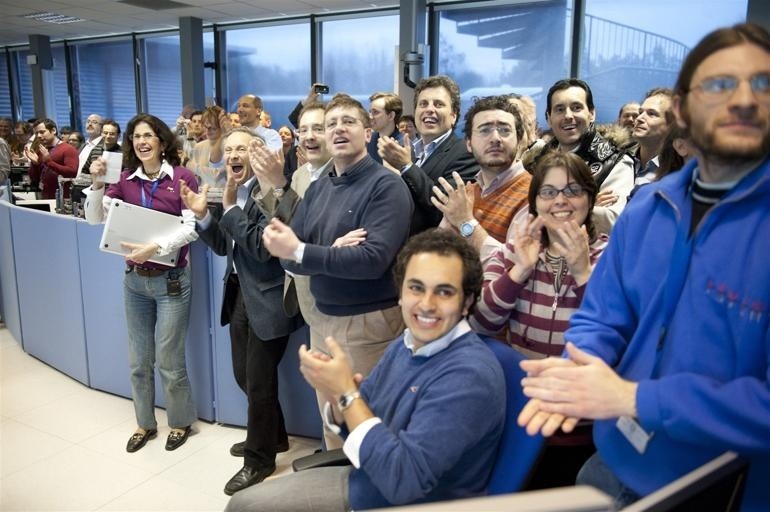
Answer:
[92,185,102,190]
[187,138,194,140]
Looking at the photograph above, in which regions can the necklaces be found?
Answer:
[143,170,160,179]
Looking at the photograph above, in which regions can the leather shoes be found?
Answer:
[165,425,191,452]
[126,427,158,452]
[225,465,275,496]
[231,437,289,457]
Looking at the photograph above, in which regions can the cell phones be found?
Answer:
[179,119,190,134]
[315,85,330,94]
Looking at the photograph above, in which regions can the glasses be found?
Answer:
[689,72,770,98]
[537,187,586,199]
[129,133,158,140]
[471,127,516,135]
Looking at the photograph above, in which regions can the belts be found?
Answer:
[130,265,169,277]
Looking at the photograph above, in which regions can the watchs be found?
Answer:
[294,242,306,264]
[152,242,161,254]
[274,180,292,197]
[460,219,479,238]
[399,164,407,172]
[337,391,361,413]
[28,139,33,143]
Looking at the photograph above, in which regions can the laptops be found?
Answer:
[99,198,185,267]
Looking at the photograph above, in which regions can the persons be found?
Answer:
[68,132,86,151]
[76,115,103,175]
[288,83,323,129]
[525,79,635,236]
[60,126,72,142]
[468,152,610,491]
[283,146,298,191]
[82,120,128,174]
[190,112,207,142]
[262,93,414,454]
[225,229,506,512]
[207,95,283,188]
[259,110,271,128]
[368,93,404,165]
[0,137,11,185]
[627,90,674,184]
[619,103,640,137]
[518,23,770,512]
[540,131,554,142]
[291,104,333,199]
[184,106,229,165]
[398,115,418,144]
[377,76,480,236]
[278,125,295,157]
[170,104,195,135]
[27,119,36,131]
[177,136,227,188]
[14,121,36,163]
[431,97,533,273]
[24,119,79,199]
[82,113,198,452]
[296,145,308,167]
[627,120,695,202]
[0,118,14,143]
[179,127,305,496]
[228,112,242,128]
[508,98,547,164]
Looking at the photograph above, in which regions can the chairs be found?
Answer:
[291,339,545,495]
